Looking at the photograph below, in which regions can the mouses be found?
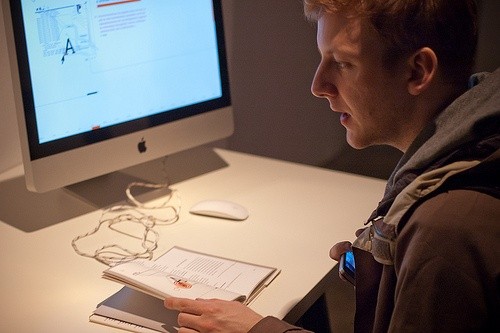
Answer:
[192,201,249,221]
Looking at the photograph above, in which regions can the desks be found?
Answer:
[0,146,392,333]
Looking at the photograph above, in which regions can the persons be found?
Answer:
[164,0,500,333]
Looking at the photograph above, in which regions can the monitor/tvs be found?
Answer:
[0,0,234,211]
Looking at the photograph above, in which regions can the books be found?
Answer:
[89,245,282,333]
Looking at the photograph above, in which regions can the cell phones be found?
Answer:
[339,252,361,285]
